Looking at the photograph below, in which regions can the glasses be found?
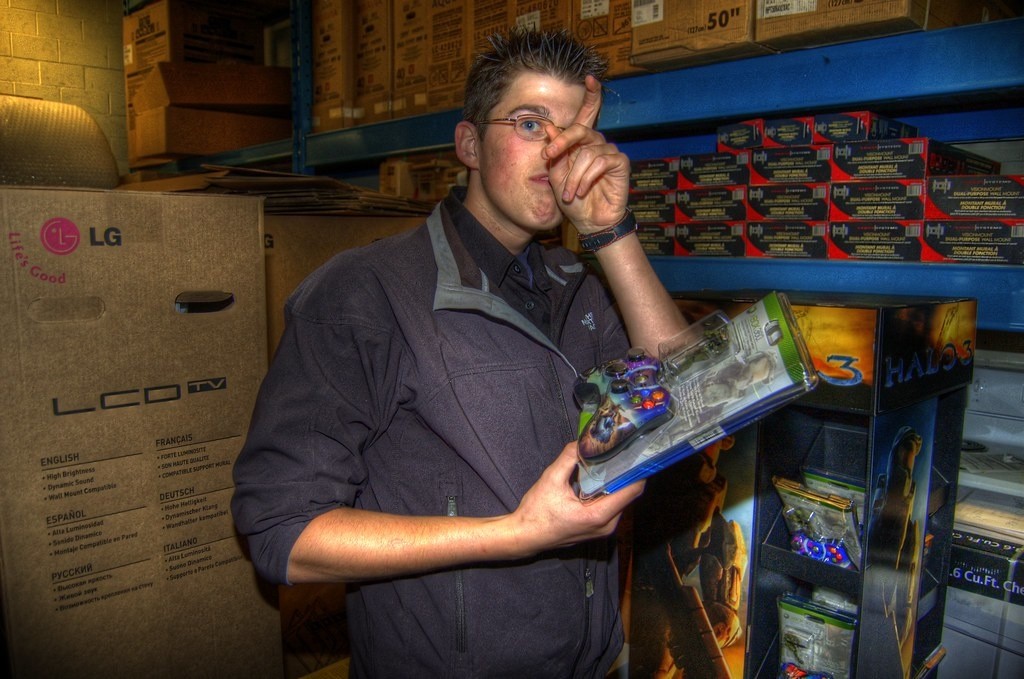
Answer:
[473,114,565,142]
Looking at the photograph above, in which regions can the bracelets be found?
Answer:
[578,206,639,253]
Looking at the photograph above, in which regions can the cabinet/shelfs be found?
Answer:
[744,291,977,679]
[165,0,1024,328]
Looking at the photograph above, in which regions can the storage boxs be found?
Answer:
[122,0,263,169]
[626,189,675,223]
[747,182,830,221]
[675,186,747,225]
[678,150,751,190]
[131,62,292,115]
[829,223,924,262]
[0,150,469,679]
[628,158,680,190]
[830,179,926,222]
[635,223,675,258]
[265,0,1018,151]
[136,106,291,159]
[923,220,1024,266]
[924,175,1024,219]
[745,221,829,259]
[675,221,745,258]
[831,137,1002,182]
[751,145,831,186]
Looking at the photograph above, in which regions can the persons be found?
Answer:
[228,20,694,679]
